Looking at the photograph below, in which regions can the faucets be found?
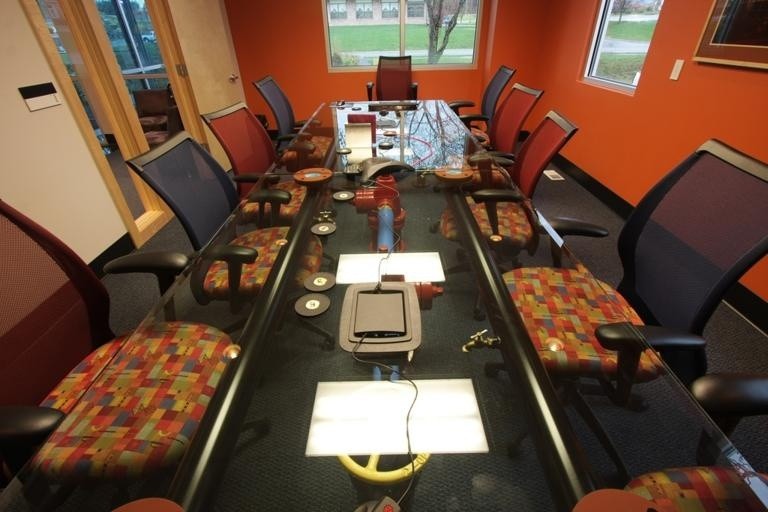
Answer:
[313,210,334,224]
[464,327,502,351]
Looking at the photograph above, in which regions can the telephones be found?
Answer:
[343,157,416,186]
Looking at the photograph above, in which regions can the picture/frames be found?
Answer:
[691,1,768,72]
